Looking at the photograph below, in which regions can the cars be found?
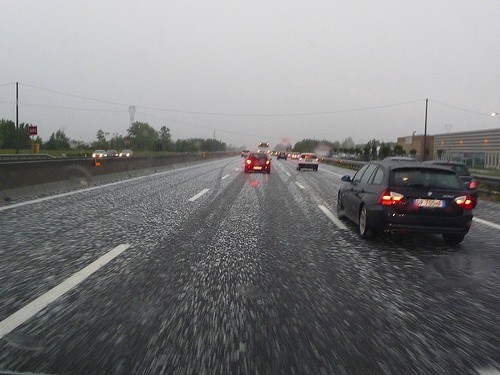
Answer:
[240,149,250,158]
[277,152,288,160]
[244,152,273,173]
[291,152,301,159]
[92,149,134,158]
[297,153,319,171]
[337,156,479,244]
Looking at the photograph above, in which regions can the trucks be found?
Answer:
[257,142,271,156]
